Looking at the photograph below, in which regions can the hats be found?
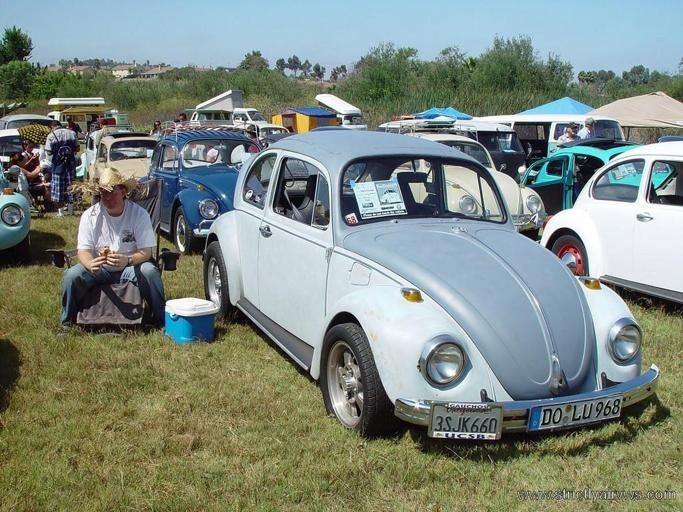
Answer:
[80,167,137,193]
[567,121,578,129]
[585,117,598,125]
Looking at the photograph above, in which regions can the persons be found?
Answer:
[150,112,222,163]
[248,124,263,153]
[557,118,597,143]
[0,116,82,216]
[60,186,166,331]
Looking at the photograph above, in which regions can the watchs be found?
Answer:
[128,256,133,266]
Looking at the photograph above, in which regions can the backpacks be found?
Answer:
[52,131,72,166]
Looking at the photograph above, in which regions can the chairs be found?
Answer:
[289,174,330,224]
[658,195,683,206]
[45,175,179,333]
[3,172,46,218]
